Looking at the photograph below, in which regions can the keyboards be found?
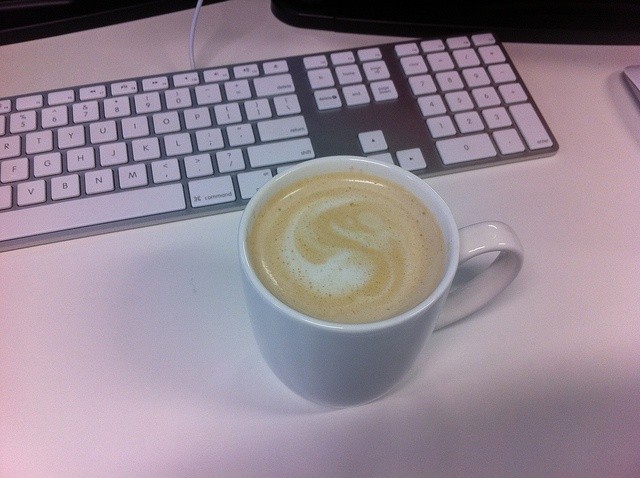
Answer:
[0,31,559,254]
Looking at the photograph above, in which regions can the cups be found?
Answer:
[239,154,523,409]
